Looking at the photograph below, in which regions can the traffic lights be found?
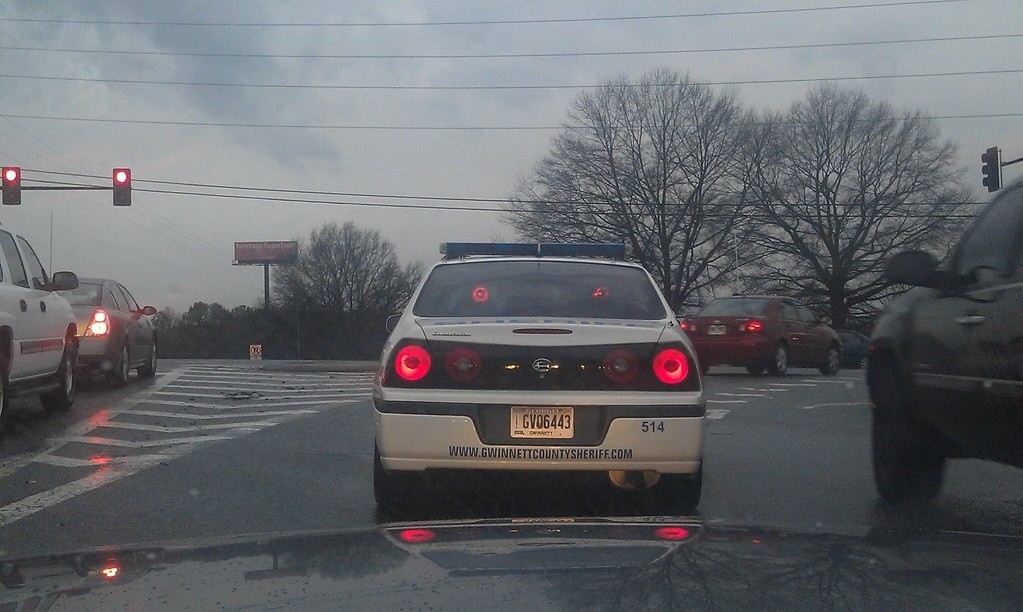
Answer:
[2,167,21,204]
[113,169,132,207]
[981,146,1004,191]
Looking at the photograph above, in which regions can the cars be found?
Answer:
[681,296,843,377]
[836,328,869,370]
[371,242,706,512]
[58,277,158,387]
[866,175,1023,503]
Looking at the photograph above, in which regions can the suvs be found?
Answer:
[0,229,79,435]
